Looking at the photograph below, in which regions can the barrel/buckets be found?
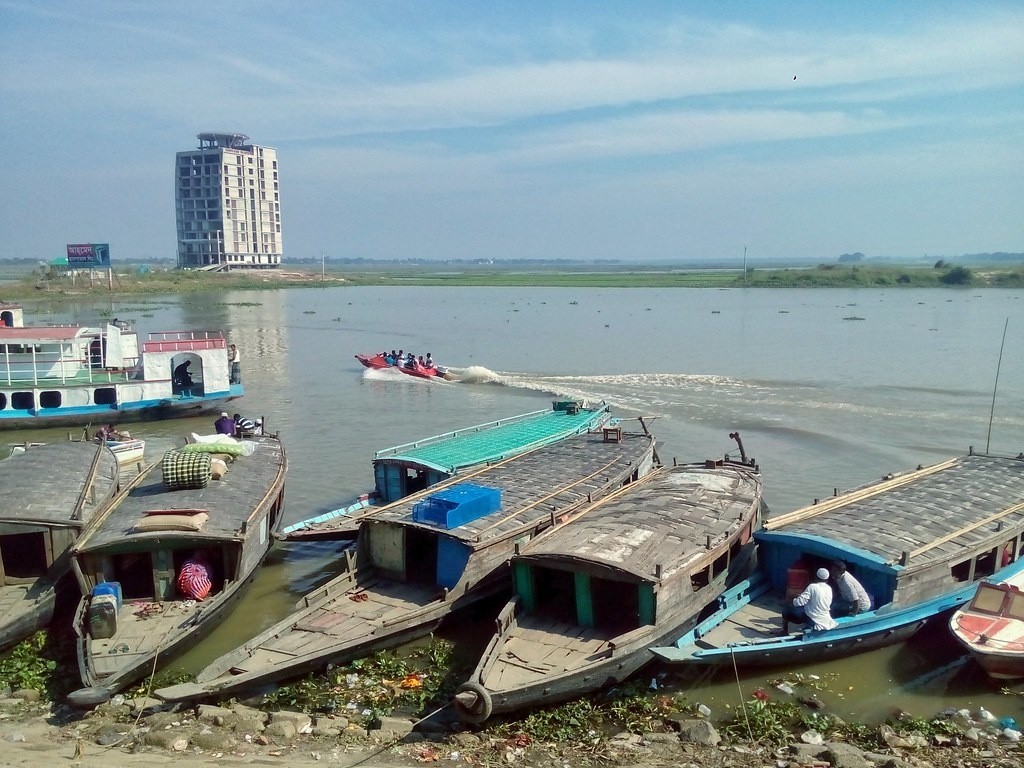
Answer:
[88,582,122,640]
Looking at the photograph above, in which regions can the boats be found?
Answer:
[354,353,449,380]
[455,433,773,719]
[947,554,1024,681]
[273,398,621,543]
[152,416,657,705]
[0,425,121,655]
[8,431,145,464]
[648,446,1024,670]
[0,305,245,428]
[66,417,290,705]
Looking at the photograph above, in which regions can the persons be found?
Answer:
[96,423,118,441]
[214,412,262,438]
[174,360,193,386]
[229,344,240,384]
[774,568,839,637]
[830,561,871,618]
[383,350,437,375]
[975,545,1009,573]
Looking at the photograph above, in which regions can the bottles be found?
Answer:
[695,701,711,716]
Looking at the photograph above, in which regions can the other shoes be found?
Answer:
[774,631,789,636]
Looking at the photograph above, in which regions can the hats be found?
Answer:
[817,568,830,579]
[256,419,262,424]
[221,412,228,416]
